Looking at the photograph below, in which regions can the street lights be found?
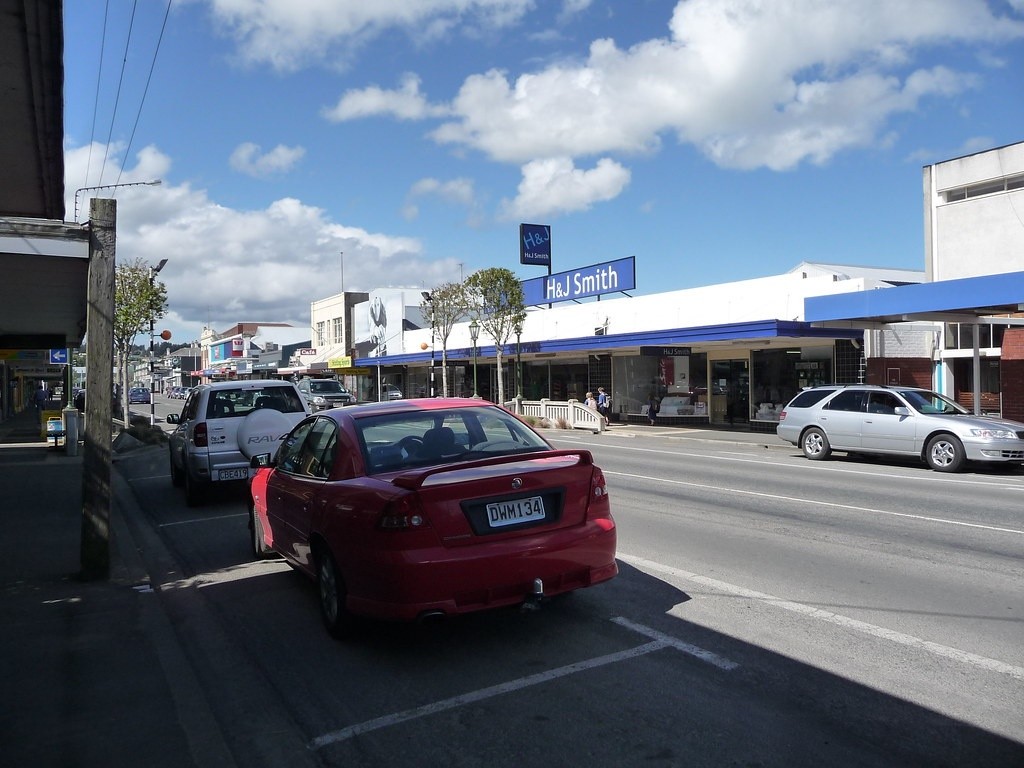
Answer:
[469,318,481,398]
[149,259,171,432]
[74,179,162,222]
[420,290,437,398]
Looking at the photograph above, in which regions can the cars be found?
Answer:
[73,388,85,413]
[128,388,151,404]
[167,386,253,405]
[631,380,728,395]
[777,383,1024,473]
[247,398,619,638]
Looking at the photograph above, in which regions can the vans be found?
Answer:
[379,383,402,401]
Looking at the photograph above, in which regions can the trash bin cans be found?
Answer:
[62,409,80,457]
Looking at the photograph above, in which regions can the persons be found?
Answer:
[585,392,595,405]
[648,394,656,425]
[35,386,53,424]
[598,387,609,426]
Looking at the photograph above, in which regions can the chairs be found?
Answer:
[263,397,288,411]
[415,427,455,458]
[214,399,235,412]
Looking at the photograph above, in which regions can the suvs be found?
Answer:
[167,380,315,508]
[291,379,357,414]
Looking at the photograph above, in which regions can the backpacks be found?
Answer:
[586,398,597,411]
[601,393,612,408]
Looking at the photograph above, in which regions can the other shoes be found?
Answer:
[651,420,654,426]
[606,420,609,426]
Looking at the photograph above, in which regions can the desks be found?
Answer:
[698,394,727,420]
